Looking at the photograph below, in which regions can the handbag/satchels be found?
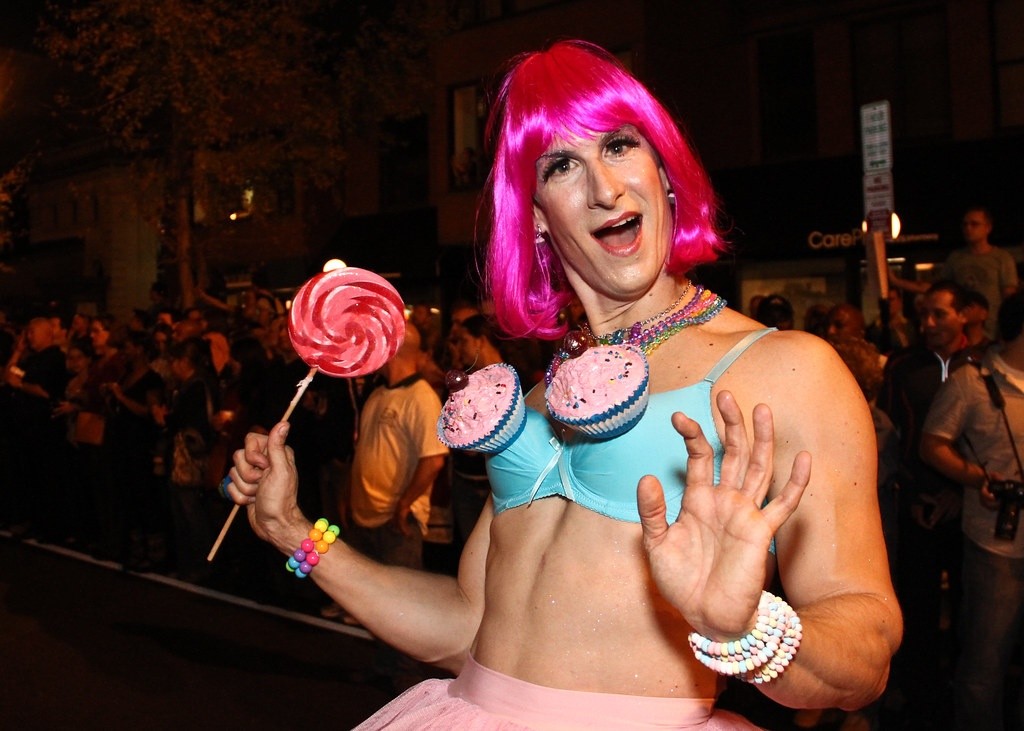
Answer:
[173,430,211,487]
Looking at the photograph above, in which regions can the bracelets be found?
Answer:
[678,589,804,687]
[297,525,340,579]
[284,515,328,572]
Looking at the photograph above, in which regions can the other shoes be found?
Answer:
[321,605,361,624]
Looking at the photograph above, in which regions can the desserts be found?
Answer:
[544,344,651,439]
[435,355,527,454]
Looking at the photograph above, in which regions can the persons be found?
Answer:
[222,40,904,730]
[1,260,527,626]
[747,208,1021,731]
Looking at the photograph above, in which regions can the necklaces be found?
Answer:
[544,278,727,395]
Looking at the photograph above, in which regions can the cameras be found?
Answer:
[988,479,1024,540]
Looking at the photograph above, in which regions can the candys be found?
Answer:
[288,266,407,380]
